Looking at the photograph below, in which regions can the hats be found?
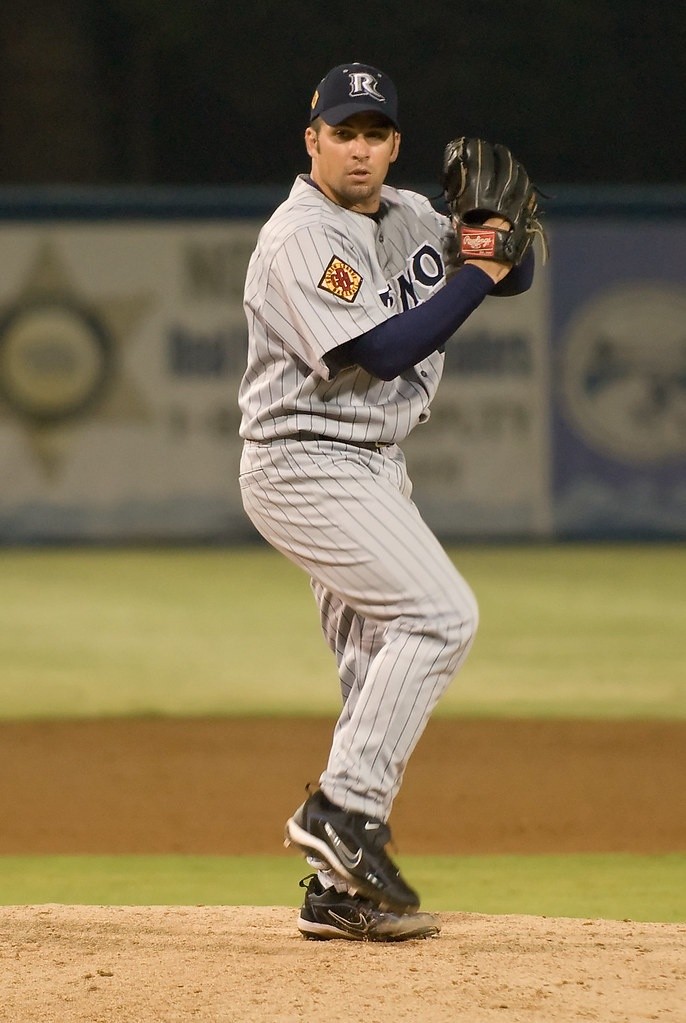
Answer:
[310,62,399,130]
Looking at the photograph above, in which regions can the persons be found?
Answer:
[237,61,539,942]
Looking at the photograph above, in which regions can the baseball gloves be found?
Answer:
[443,137,548,267]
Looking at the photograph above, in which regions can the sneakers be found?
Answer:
[282,781,420,915]
[297,871,441,942]
